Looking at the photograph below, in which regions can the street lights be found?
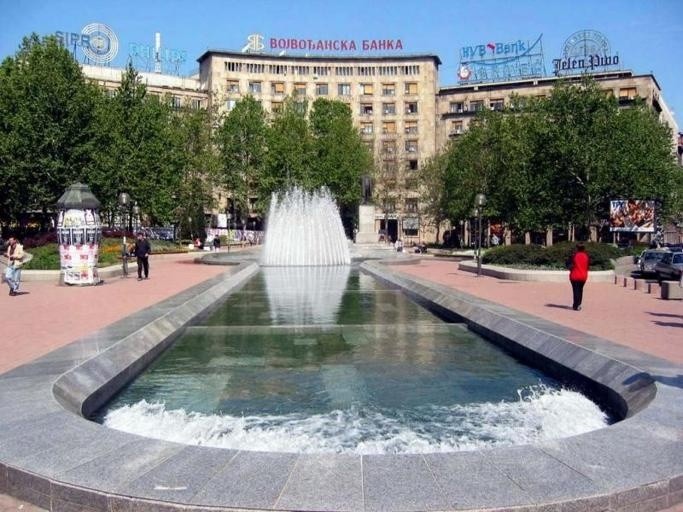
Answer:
[117,190,131,279]
[458,217,465,249]
[131,200,141,238]
[473,188,488,278]
[226,210,232,254]
[253,220,257,246]
[240,217,246,250]
[471,208,479,263]
[170,191,178,241]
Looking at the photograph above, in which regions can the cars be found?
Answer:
[619,237,632,248]
[99,225,175,241]
[635,238,683,281]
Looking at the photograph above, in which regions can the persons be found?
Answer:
[569,243,589,310]
[213,234,220,252]
[133,232,150,281]
[3,237,32,296]
